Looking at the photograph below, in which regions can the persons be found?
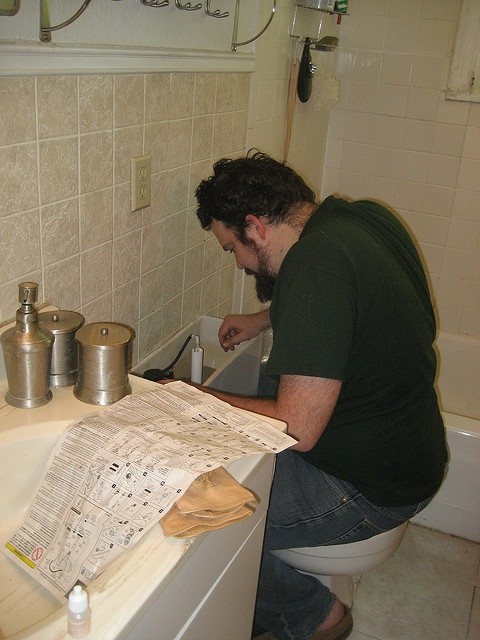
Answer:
[148,144,451,639]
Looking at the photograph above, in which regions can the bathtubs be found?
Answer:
[409,330,479,435]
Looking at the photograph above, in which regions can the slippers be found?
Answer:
[252,597,354,640]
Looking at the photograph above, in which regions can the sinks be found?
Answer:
[0,420,162,639]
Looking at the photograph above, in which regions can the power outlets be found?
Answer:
[131,154,151,211]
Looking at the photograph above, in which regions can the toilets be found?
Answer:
[127,315,410,609]
